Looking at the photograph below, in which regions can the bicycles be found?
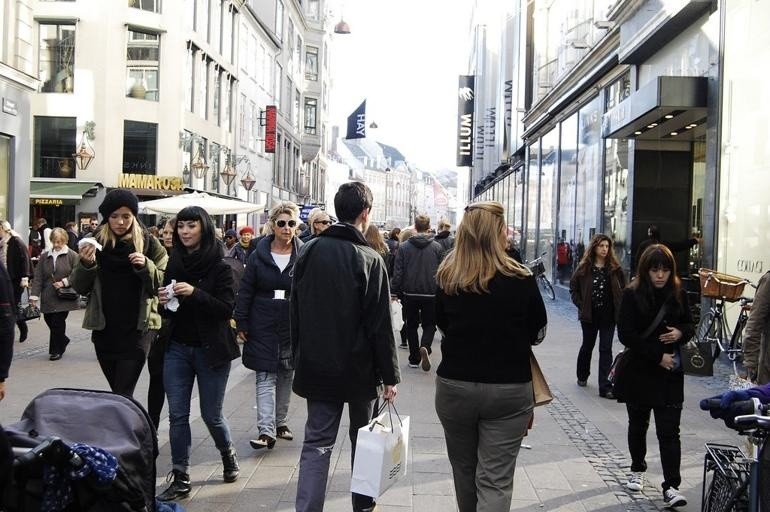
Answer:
[694,267,758,383]
[701,397,770,512]
[522,252,555,301]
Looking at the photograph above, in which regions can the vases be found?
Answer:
[131,83,146,99]
[58,158,73,178]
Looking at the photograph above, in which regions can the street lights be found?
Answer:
[385,168,390,222]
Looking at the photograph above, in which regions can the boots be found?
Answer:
[154,465,192,503]
[221,450,240,482]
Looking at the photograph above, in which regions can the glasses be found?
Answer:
[273,219,297,228]
[314,220,333,225]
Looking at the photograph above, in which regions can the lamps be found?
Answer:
[334,20,350,33]
[72,121,95,170]
[233,154,256,190]
[211,143,238,185]
[180,131,210,179]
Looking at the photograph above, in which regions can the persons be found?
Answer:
[0,219,35,343]
[28,227,79,361]
[434,200,548,512]
[297,207,322,238]
[399,227,416,349]
[385,227,401,257]
[741,270,770,387]
[390,215,446,372]
[235,201,305,450]
[79,217,99,241]
[504,235,523,265]
[434,221,455,252]
[68,188,169,397]
[146,217,178,431]
[65,222,79,253]
[552,236,576,286]
[290,181,402,512]
[569,232,626,400]
[229,226,255,268]
[224,228,239,257]
[364,224,390,270]
[634,223,703,267]
[32,218,53,257]
[615,242,695,508]
[302,211,333,243]
[0,260,18,401]
[156,205,241,503]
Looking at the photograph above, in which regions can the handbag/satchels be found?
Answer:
[529,346,553,407]
[606,301,667,389]
[350,396,410,500]
[51,273,80,300]
[17,286,40,321]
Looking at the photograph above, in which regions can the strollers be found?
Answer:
[1,387,185,511]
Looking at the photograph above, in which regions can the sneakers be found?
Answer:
[19,328,28,342]
[408,362,419,368]
[664,487,687,508]
[604,392,614,399]
[626,472,644,491]
[577,379,588,387]
[420,346,431,372]
[399,344,407,349]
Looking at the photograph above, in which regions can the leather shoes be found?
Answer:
[51,353,62,360]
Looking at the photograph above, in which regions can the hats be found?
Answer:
[226,230,238,241]
[240,228,254,235]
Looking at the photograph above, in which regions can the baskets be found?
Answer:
[699,268,745,299]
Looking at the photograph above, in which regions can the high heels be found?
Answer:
[250,435,277,449]
[280,431,293,440]
